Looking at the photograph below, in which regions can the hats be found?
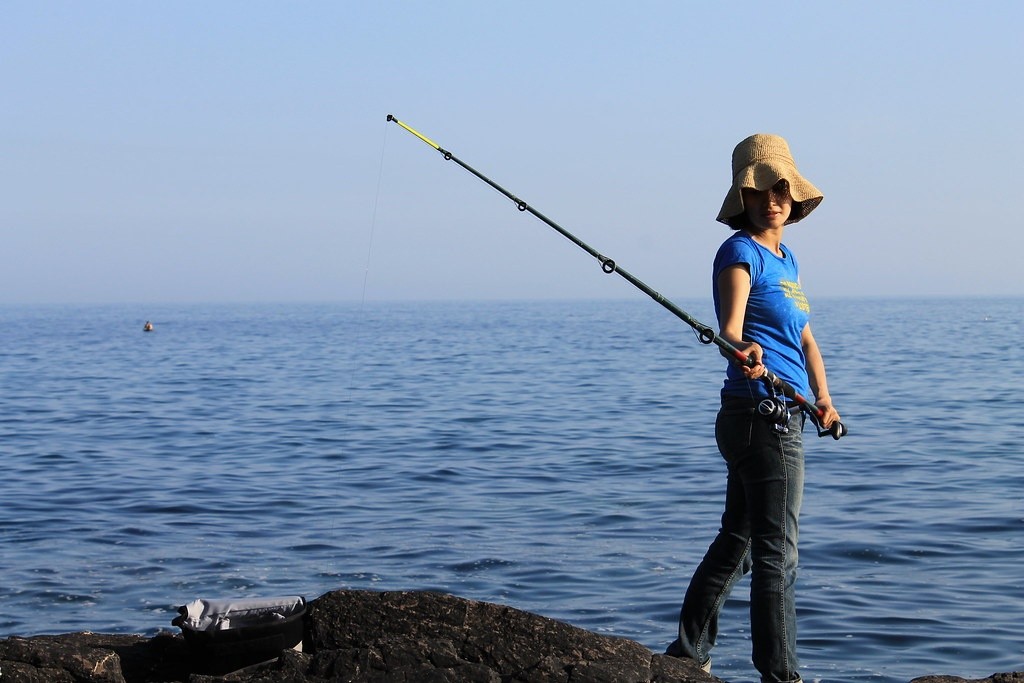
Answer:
[715,133,824,231]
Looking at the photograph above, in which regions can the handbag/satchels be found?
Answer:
[171,596,309,640]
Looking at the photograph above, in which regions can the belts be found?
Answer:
[787,404,803,415]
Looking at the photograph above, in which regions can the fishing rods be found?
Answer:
[386,113,849,441]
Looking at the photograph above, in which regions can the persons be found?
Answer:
[659,133,841,683]
